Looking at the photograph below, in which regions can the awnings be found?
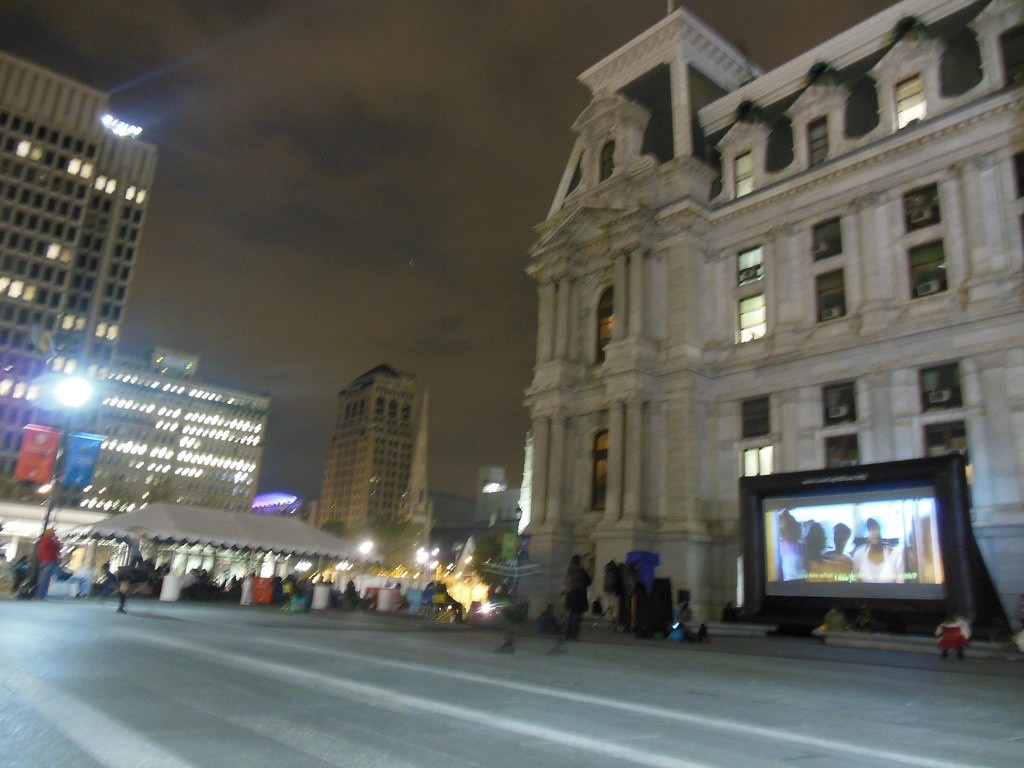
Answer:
[61,503,383,565]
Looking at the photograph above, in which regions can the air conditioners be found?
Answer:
[742,267,758,279]
[912,209,931,223]
[840,460,858,468]
[917,280,940,296]
[821,307,841,321]
[929,389,951,403]
[810,242,828,256]
[946,448,965,456]
[829,406,847,417]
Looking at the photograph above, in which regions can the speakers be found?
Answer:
[603,561,620,594]
[677,590,690,603]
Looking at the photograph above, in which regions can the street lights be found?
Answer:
[20,375,94,598]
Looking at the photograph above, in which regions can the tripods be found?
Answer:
[592,594,616,629]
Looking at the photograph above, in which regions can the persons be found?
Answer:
[591,597,602,615]
[566,554,592,641]
[720,602,735,624]
[537,604,559,633]
[935,605,971,664]
[778,515,904,583]
[7,555,463,623]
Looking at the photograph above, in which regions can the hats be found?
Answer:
[45,528,56,536]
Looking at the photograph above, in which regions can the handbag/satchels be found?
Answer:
[557,574,572,595]
